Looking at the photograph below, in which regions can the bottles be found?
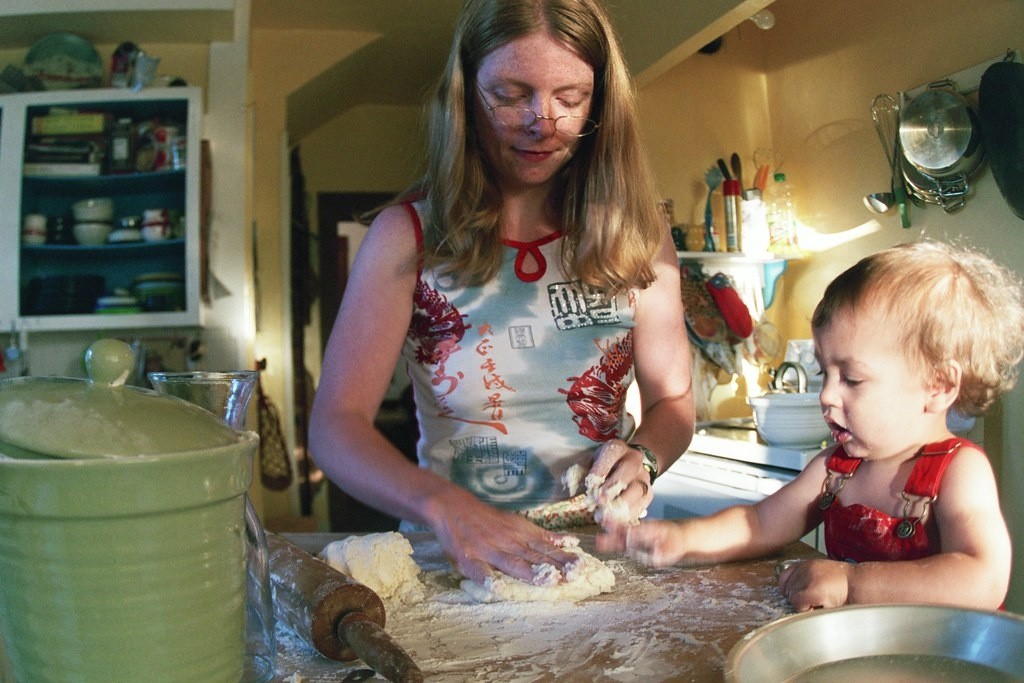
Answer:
[146,369,276,682]
[769,172,798,257]
[722,180,742,254]
[110,117,136,175]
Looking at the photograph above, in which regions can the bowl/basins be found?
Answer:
[71,197,114,223]
[722,602,1024,683]
[749,393,833,450]
[72,223,111,244]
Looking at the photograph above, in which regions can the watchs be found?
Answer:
[628,444,657,485]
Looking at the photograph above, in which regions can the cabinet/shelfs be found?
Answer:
[0,84,202,333]
[635,415,851,565]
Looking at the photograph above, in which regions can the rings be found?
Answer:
[638,480,648,495]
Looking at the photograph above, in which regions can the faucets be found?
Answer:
[773,361,809,394]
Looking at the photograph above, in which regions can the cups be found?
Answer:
[0,339,259,683]
[740,189,769,253]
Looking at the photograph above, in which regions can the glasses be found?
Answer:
[472,81,602,138]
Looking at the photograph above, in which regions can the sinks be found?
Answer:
[694,415,763,445]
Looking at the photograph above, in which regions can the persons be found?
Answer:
[595,242,1024,614]
[306,0,696,588]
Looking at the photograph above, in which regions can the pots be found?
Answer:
[898,52,1024,220]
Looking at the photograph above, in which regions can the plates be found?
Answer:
[25,33,104,87]
